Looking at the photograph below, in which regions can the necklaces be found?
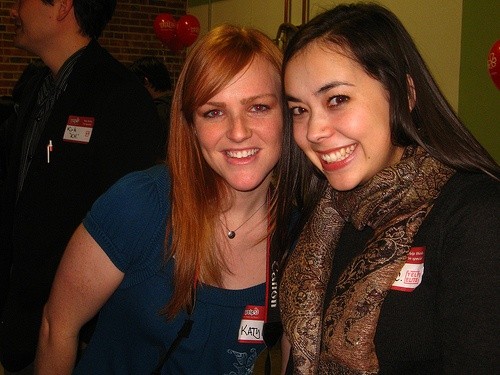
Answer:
[212,202,267,239]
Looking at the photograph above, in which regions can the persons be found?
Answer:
[36,24,289,375]
[0,0,194,375]
[281,1,500,375]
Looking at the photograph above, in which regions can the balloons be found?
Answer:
[150,13,199,53]
[489,39,500,93]
